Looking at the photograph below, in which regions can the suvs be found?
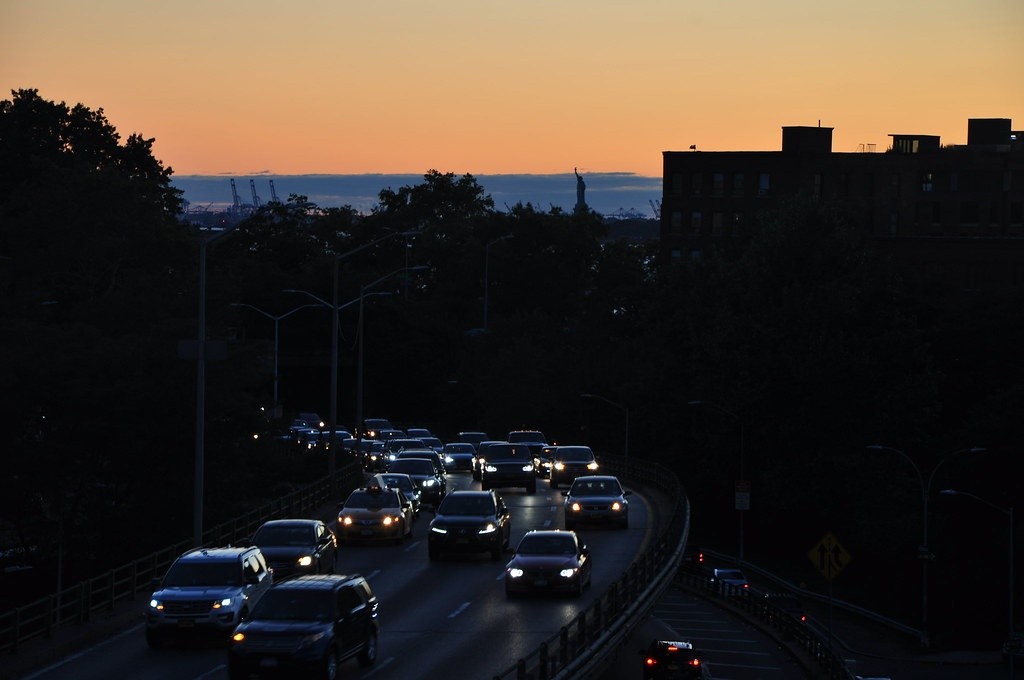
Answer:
[456,432,537,494]
[225,572,380,680]
[428,488,511,560]
[385,450,444,499]
[145,544,273,647]
[540,445,600,487]
[508,429,548,461]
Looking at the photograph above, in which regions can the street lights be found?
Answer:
[328,230,424,460]
[484,235,514,332]
[580,393,629,474]
[230,301,329,424]
[193,203,317,549]
[865,445,1015,680]
[687,400,745,561]
[282,264,429,448]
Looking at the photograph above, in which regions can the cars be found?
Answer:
[562,476,632,528]
[244,487,414,577]
[284,412,487,472]
[368,474,420,517]
[505,529,592,600]
